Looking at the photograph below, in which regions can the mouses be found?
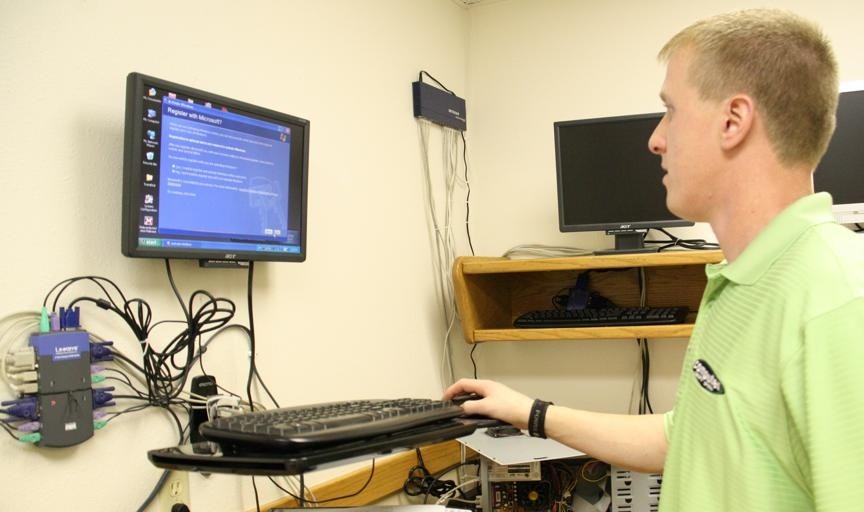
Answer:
[451,394,488,419]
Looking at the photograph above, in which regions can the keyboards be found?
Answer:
[514,306,686,329]
[197,398,462,450]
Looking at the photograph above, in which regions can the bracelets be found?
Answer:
[525,397,555,441]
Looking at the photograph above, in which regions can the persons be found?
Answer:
[438,3,864,510]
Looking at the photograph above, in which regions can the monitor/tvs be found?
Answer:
[121,71,310,268]
[553,112,695,255]
[810,83,863,224]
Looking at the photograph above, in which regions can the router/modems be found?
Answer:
[411,79,466,132]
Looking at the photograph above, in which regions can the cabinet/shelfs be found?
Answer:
[449,247,727,343]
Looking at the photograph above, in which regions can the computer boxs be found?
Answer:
[479,450,662,512]
